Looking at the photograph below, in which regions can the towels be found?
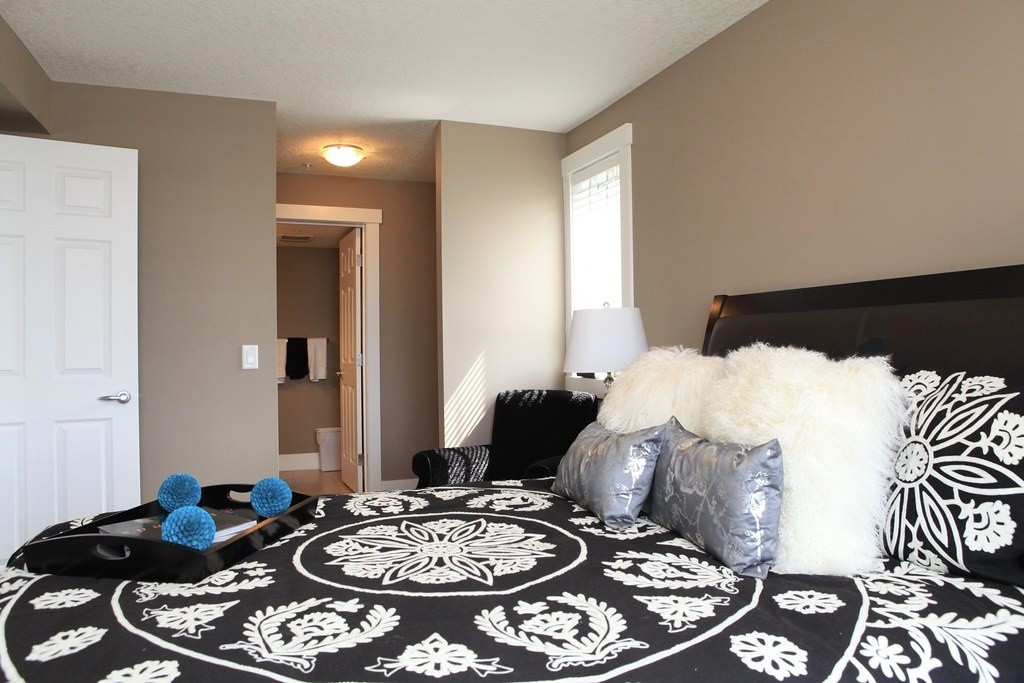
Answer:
[277,338,288,384]
[285,337,308,380]
[307,337,327,382]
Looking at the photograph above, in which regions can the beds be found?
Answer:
[0,264,1024,683]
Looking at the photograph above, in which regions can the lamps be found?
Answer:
[561,302,651,388]
[320,143,366,169]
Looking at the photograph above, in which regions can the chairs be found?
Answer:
[411,389,599,492]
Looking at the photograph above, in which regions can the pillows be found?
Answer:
[550,419,666,531]
[650,414,785,581]
